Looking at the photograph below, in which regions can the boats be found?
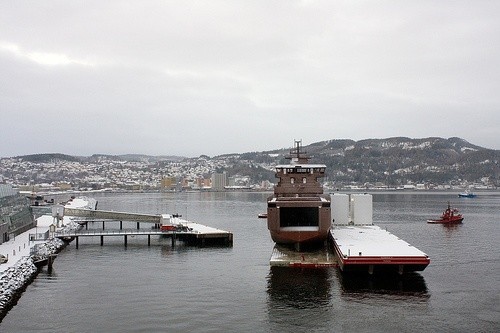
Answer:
[457,190,477,199]
[265,137,333,247]
[426,199,465,224]
